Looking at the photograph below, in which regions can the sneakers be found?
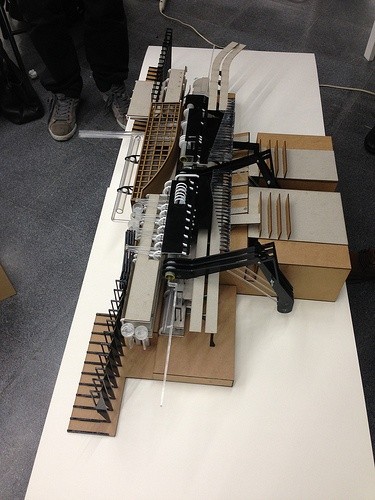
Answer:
[99,82,130,130]
[46,93,79,141]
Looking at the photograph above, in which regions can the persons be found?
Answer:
[16,0,131,140]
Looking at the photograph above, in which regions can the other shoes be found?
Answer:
[364,126,375,155]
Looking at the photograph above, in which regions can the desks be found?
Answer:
[25,46,374,499]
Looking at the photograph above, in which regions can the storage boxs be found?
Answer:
[219,187,351,303]
[153,284,236,387]
[254,132,339,191]
